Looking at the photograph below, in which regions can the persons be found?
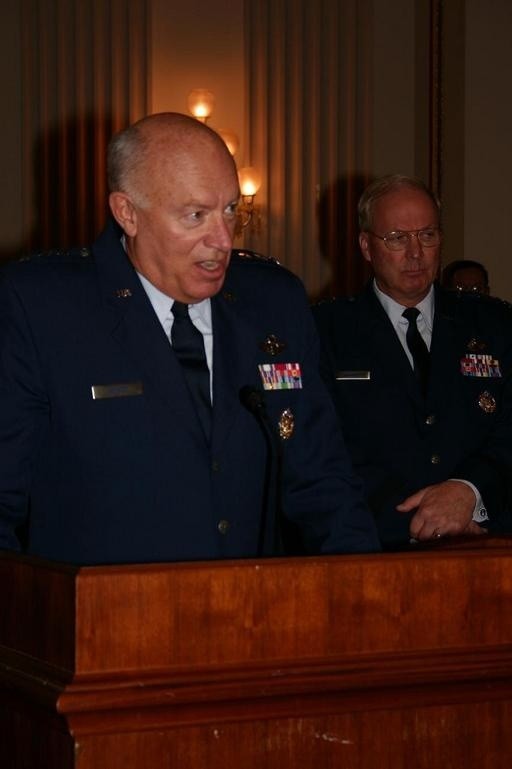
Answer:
[438,258,491,298]
[3,112,384,563]
[318,172,511,551]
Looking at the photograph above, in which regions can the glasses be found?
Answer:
[449,282,490,297]
[367,227,444,252]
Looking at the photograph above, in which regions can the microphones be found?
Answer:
[239,380,287,565]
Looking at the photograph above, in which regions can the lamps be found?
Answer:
[186,86,265,242]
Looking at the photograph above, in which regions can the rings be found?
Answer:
[434,531,444,538]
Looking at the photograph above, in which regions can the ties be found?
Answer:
[170,299,214,450]
[400,307,433,407]
